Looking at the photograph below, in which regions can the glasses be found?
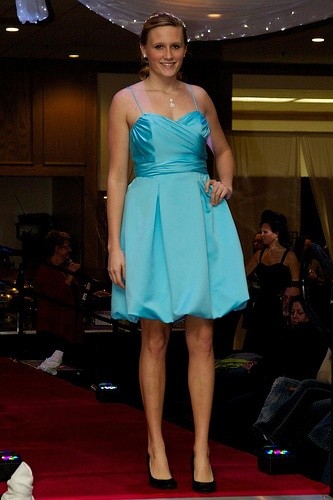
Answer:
[62,246,69,250]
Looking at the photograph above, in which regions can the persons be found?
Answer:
[105,10,250,495]
[34,232,85,327]
[237,210,332,478]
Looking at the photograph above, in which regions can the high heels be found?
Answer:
[146,454,177,489]
[191,449,216,492]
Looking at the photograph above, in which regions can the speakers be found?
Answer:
[180,59,233,133]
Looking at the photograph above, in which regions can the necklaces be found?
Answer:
[150,75,181,111]
